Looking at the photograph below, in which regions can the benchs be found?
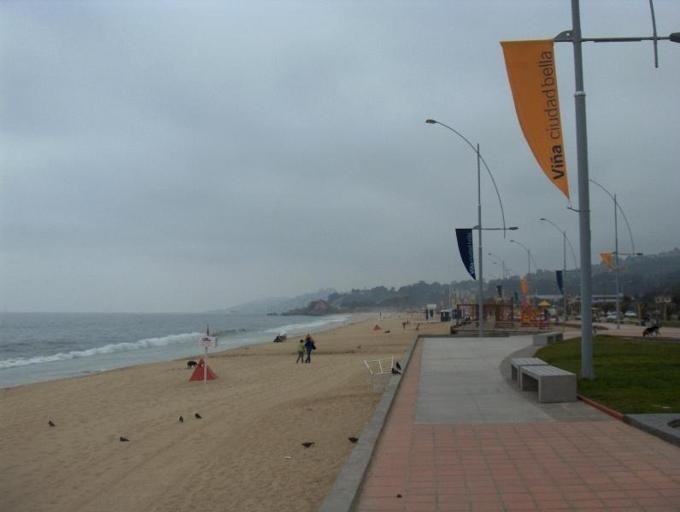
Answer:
[508,355,578,404]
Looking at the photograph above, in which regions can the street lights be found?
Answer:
[485,178,644,329]
[550,0,679,380]
[422,117,522,336]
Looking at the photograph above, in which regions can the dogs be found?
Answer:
[187,361,197,368]
[642,327,659,336]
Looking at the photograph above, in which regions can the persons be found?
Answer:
[295,338,305,363]
[303,333,316,363]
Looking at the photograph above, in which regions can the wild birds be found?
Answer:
[195,413,202,419]
[302,442,315,448]
[349,437,360,443]
[48,420,55,426]
[392,368,401,376]
[178,416,183,422]
[396,362,401,370]
[120,436,129,441]
